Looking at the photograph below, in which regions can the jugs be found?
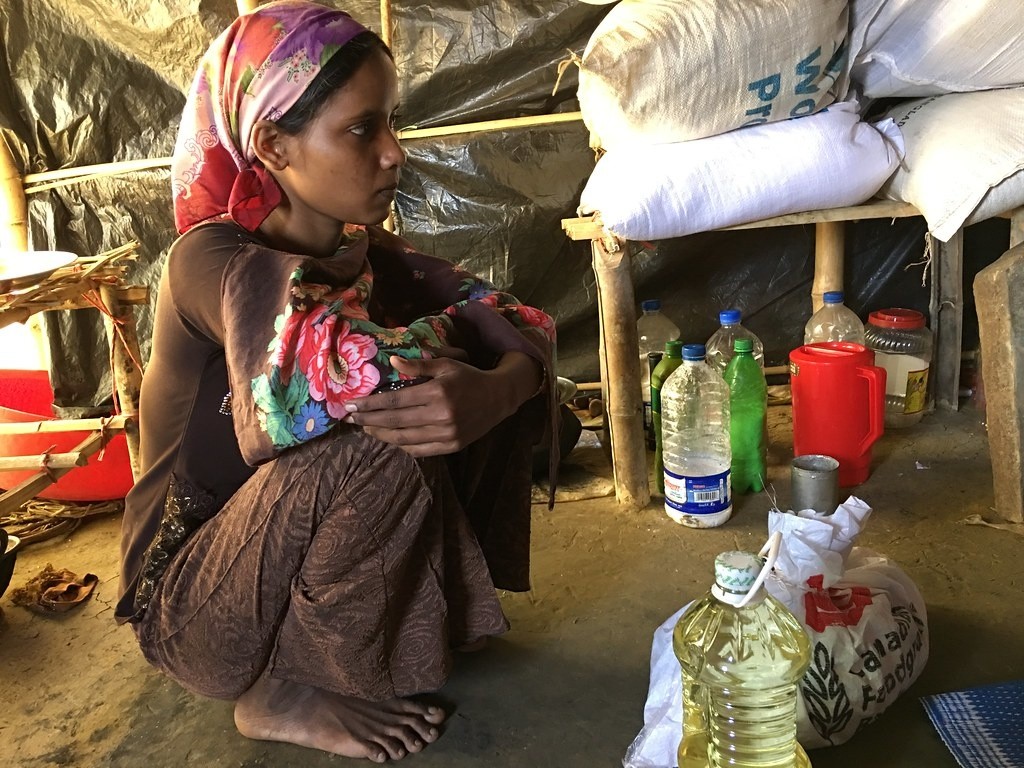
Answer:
[789,341,887,486]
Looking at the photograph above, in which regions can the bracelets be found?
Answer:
[530,366,548,399]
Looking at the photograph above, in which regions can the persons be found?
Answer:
[115,0,558,762]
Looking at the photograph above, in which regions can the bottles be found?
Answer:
[633,299,680,452]
[703,308,764,388]
[663,344,733,528]
[651,340,689,495]
[725,339,767,494]
[802,293,865,348]
[667,545,816,768]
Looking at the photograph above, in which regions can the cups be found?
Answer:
[790,454,840,516]
